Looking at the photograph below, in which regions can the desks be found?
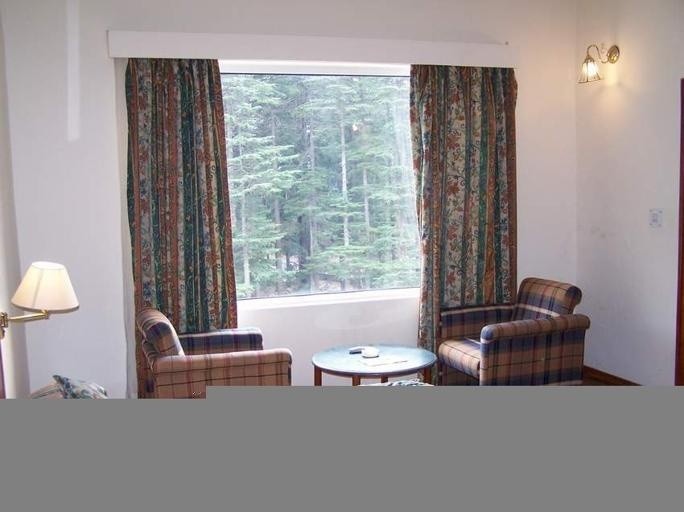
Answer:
[311,344,438,385]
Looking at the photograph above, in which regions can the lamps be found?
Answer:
[0,260,80,340]
[576,42,621,84]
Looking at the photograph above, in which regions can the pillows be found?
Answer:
[52,372,108,398]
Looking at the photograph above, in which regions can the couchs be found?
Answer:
[437,277,590,386]
[134,307,292,398]
